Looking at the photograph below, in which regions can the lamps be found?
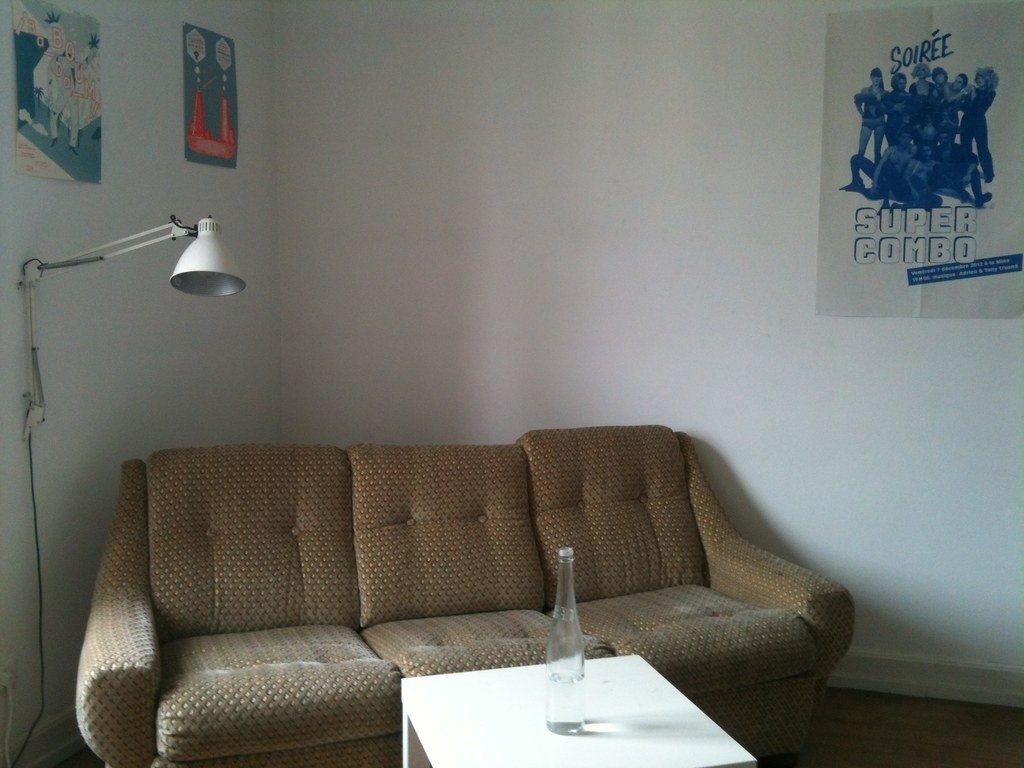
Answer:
[22,215,246,447]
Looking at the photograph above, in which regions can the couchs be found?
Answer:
[75,426,855,768]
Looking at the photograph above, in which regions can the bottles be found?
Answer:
[545,546,584,735]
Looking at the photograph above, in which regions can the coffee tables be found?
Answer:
[401,655,758,768]
